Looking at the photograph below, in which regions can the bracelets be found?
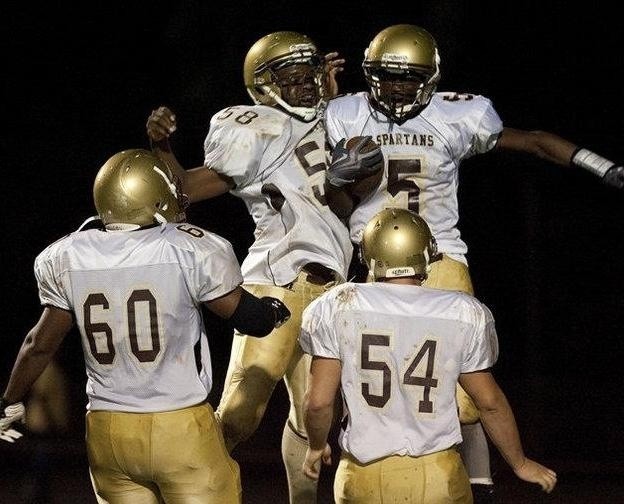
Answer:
[569,144,617,180]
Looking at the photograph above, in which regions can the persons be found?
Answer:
[0,143,292,504]
[295,207,561,504]
[142,29,347,502]
[314,23,621,504]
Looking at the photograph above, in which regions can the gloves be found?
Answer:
[259,294,291,328]
[600,166,624,193]
[323,136,386,189]
[0,397,28,445]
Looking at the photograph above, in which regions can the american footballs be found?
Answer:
[332,137,384,201]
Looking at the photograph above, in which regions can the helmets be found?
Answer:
[359,207,438,283]
[361,25,442,127]
[92,149,189,234]
[242,31,333,122]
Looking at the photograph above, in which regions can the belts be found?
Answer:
[303,264,340,285]
[430,253,449,266]
